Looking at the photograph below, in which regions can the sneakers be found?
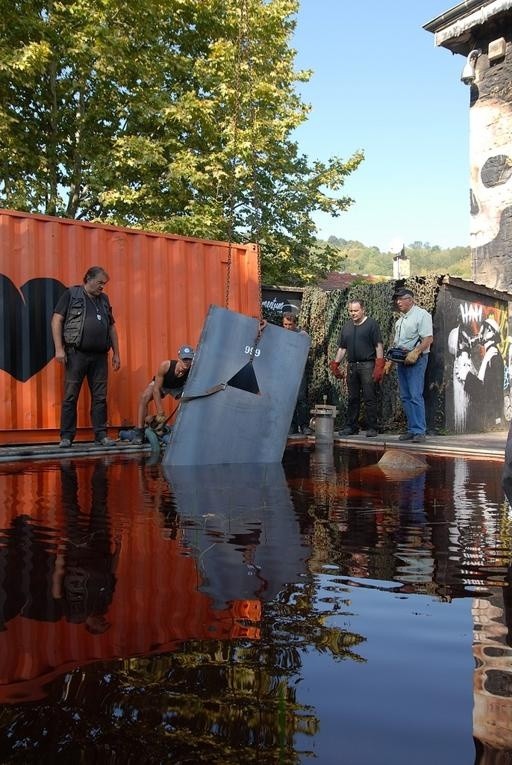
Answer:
[399,433,413,441]
[339,427,358,436]
[366,428,377,437]
[95,436,116,446]
[132,433,143,445]
[413,433,425,443]
[59,439,72,448]
[300,425,313,435]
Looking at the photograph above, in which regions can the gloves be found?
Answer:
[331,360,344,378]
[373,359,385,384]
[404,348,421,365]
[384,359,393,375]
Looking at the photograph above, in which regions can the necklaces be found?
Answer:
[84,289,102,321]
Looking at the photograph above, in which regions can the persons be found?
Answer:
[129,345,194,448]
[445,322,499,433]
[137,453,180,541]
[282,446,498,599]
[281,310,314,438]
[454,318,505,433]
[50,265,122,447]
[50,459,124,636]
[381,288,435,444]
[329,297,387,438]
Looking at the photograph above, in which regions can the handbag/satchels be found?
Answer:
[386,347,406,362]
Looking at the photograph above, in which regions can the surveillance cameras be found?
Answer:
[460,49,478,85]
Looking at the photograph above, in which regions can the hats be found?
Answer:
[180,346,194,359]
